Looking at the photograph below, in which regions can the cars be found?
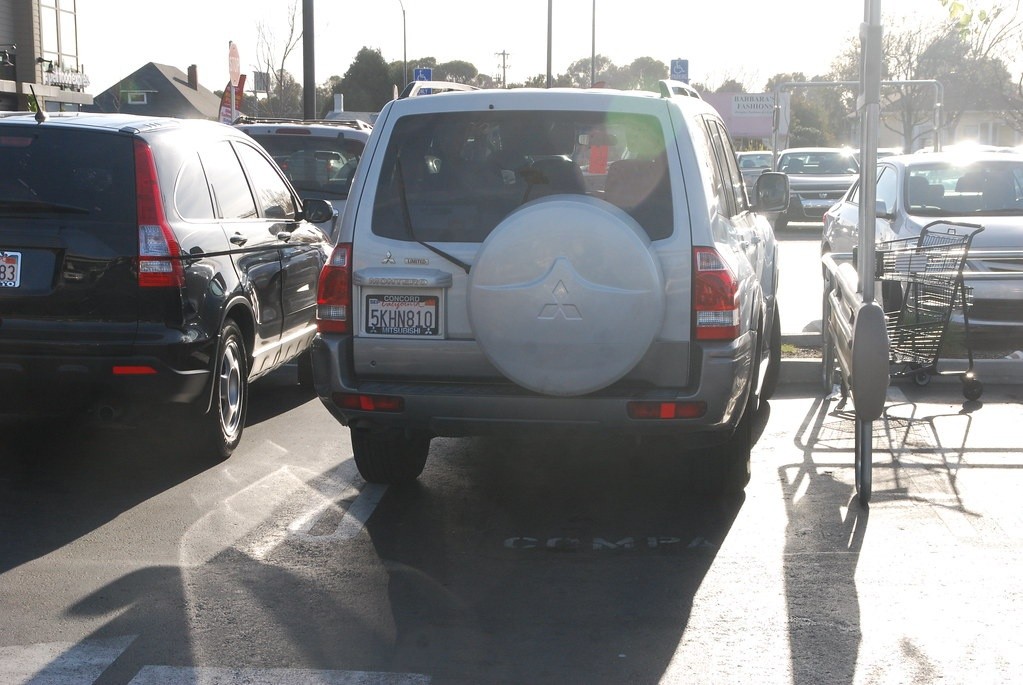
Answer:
[822,151,1023,338]
[736,150,777,198]
[775,147,859,231]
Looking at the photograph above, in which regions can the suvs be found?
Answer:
[311,79,792,498]
[0,84,337,460]
[229,115,377,246]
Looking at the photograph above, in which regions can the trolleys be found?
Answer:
[840,220,986,403]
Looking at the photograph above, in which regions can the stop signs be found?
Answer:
[228,44,241,87]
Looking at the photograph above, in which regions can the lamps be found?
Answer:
[36,57,53,73]
[0,49,14,67]
